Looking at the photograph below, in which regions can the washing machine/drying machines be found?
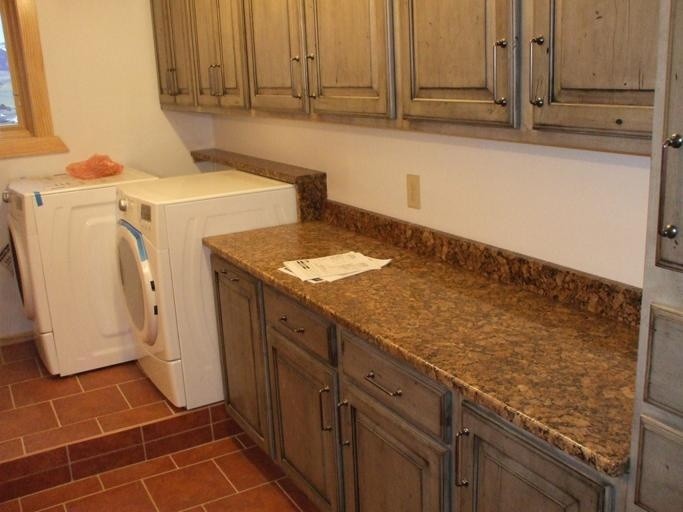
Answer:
[115,170,298,408]
[2,168,158,378]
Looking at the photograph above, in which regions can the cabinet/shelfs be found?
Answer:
[240,0,402,132]
[256,277,449,512]
[451,382,628,510]
[149,0,251,119]
[207,247,275,467]
[625,2,682,512]
[388,1,661,159]
